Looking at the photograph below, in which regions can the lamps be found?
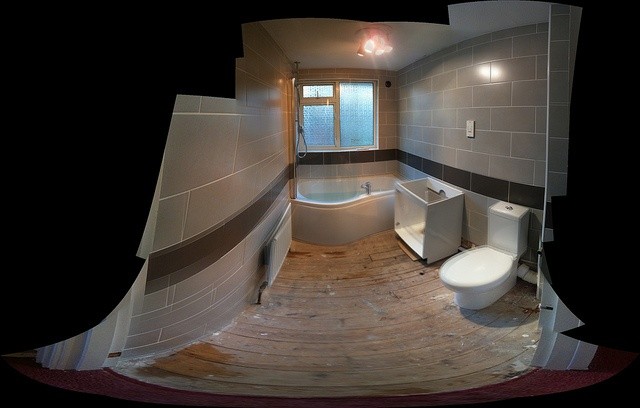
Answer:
[357,28,395,57]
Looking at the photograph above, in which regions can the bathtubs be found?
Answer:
[298,177,394,208]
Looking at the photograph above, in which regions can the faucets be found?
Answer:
[360,180,372,195]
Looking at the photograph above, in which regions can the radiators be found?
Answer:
[265,203,292,288]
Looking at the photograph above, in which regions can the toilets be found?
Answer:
[439,201,530,310]
